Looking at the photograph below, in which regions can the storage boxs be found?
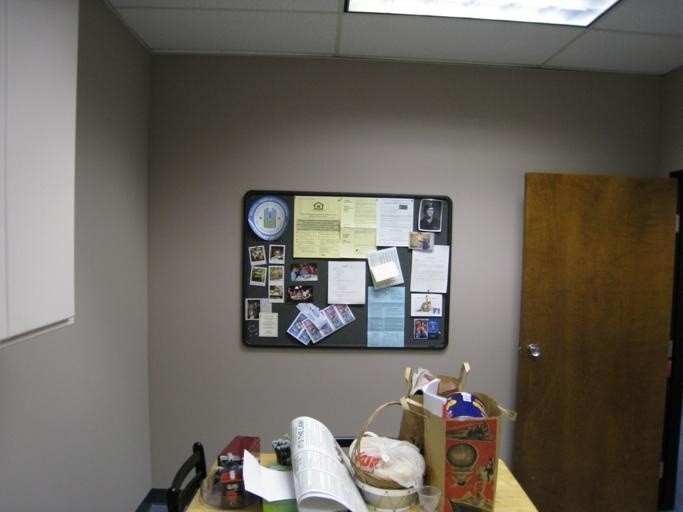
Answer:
[423,392,517,512]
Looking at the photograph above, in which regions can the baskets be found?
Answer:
[349,400,427,487]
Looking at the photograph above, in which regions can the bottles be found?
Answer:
[475,457,493,483]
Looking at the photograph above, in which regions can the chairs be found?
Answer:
[168,442,206,512]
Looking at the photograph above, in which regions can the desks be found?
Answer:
[186,452,537,512]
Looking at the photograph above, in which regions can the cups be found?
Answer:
[417,487,442,512]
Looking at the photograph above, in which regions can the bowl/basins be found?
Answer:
[199,475,254,509]
[355,479,417,511]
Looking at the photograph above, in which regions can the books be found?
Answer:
[422,375,448,418]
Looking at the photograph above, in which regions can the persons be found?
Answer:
[291,263,319,281]
[420,201,440,231]
[246,245,283,319]
